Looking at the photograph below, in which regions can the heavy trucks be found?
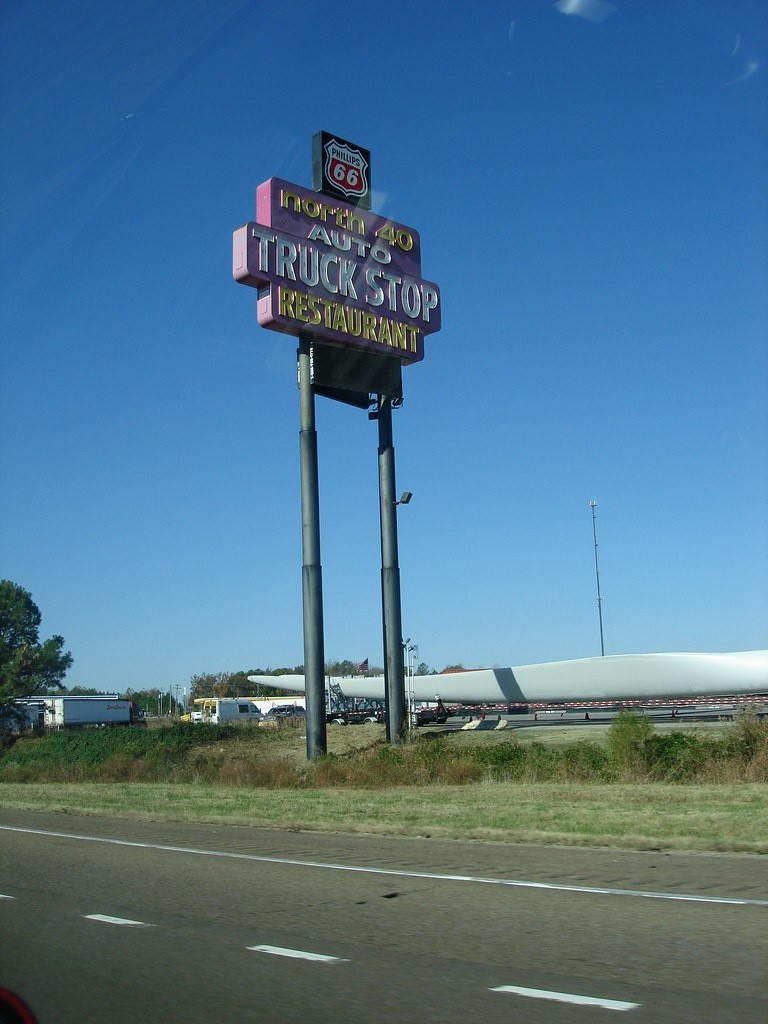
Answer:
[26,694,145,730]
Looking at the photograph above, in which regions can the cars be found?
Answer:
[263,704,306,723]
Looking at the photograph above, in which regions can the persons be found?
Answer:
[480,711,485,720]
[498,715,501,720]
[535,711,537,720]
[676,710,678,713]
[671,709,675,718]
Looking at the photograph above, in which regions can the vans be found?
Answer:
[202,699,264,727]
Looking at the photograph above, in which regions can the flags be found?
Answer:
[357,659,368,673]
